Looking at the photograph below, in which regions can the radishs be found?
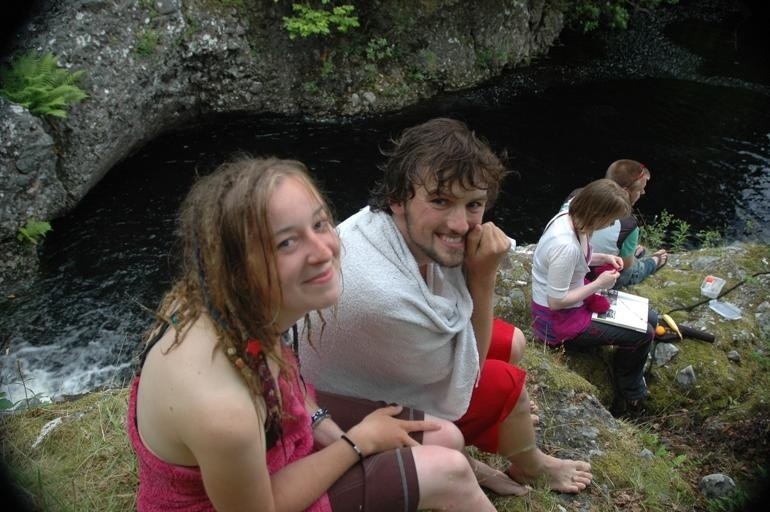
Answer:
[662,314,684,339]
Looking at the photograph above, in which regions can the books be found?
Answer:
[591,288,649,334]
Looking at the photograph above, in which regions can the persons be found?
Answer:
[530,178,658,411]
[128,155,534,511]
[559,159,668,291]
[292,117,594,494]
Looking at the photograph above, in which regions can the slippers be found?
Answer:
[634,246,645,263]
[651,250,667,270]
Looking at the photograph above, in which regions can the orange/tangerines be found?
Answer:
[656,326,665,336]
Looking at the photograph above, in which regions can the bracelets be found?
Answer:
[340,434,365,460]
[310,407,333,429]
